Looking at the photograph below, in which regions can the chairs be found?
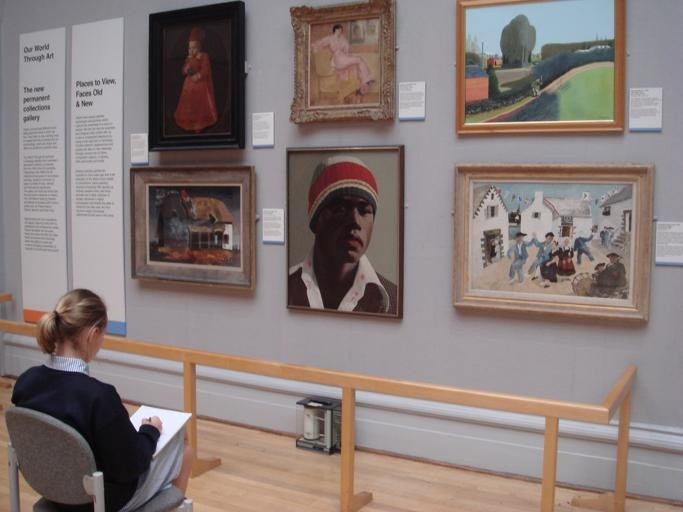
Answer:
[1,405,191,512]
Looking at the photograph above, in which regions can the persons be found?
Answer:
[311,20,380,95]
[12,288,193,512]
[486,223,628,302]
[287,156,397,314]
[173,27,219,134]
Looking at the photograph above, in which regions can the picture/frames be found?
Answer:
[142,0,247,156]
[450,162,654,326]
[283,142,407,322]
[285,0,401,127]
[450,0,624,138]
[126,163,259,294]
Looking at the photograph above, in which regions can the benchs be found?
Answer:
[309,44,359,104]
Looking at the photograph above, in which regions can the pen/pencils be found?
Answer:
[149,417,151,422]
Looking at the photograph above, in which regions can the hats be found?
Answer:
[308,155,379,233]
